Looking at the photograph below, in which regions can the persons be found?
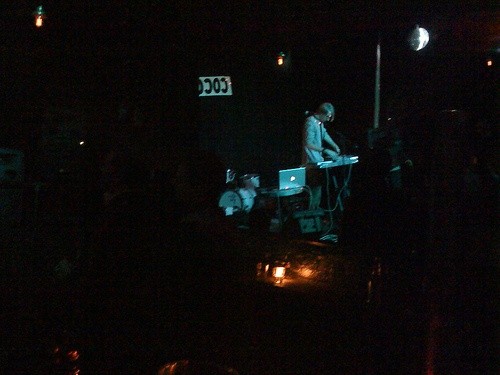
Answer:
[301,102,341,210]
[36,91,119,325]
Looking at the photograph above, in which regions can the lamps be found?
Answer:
[32,1,48,30]
[273,47,287,68]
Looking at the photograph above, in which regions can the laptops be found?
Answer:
[278,167,307,190]
